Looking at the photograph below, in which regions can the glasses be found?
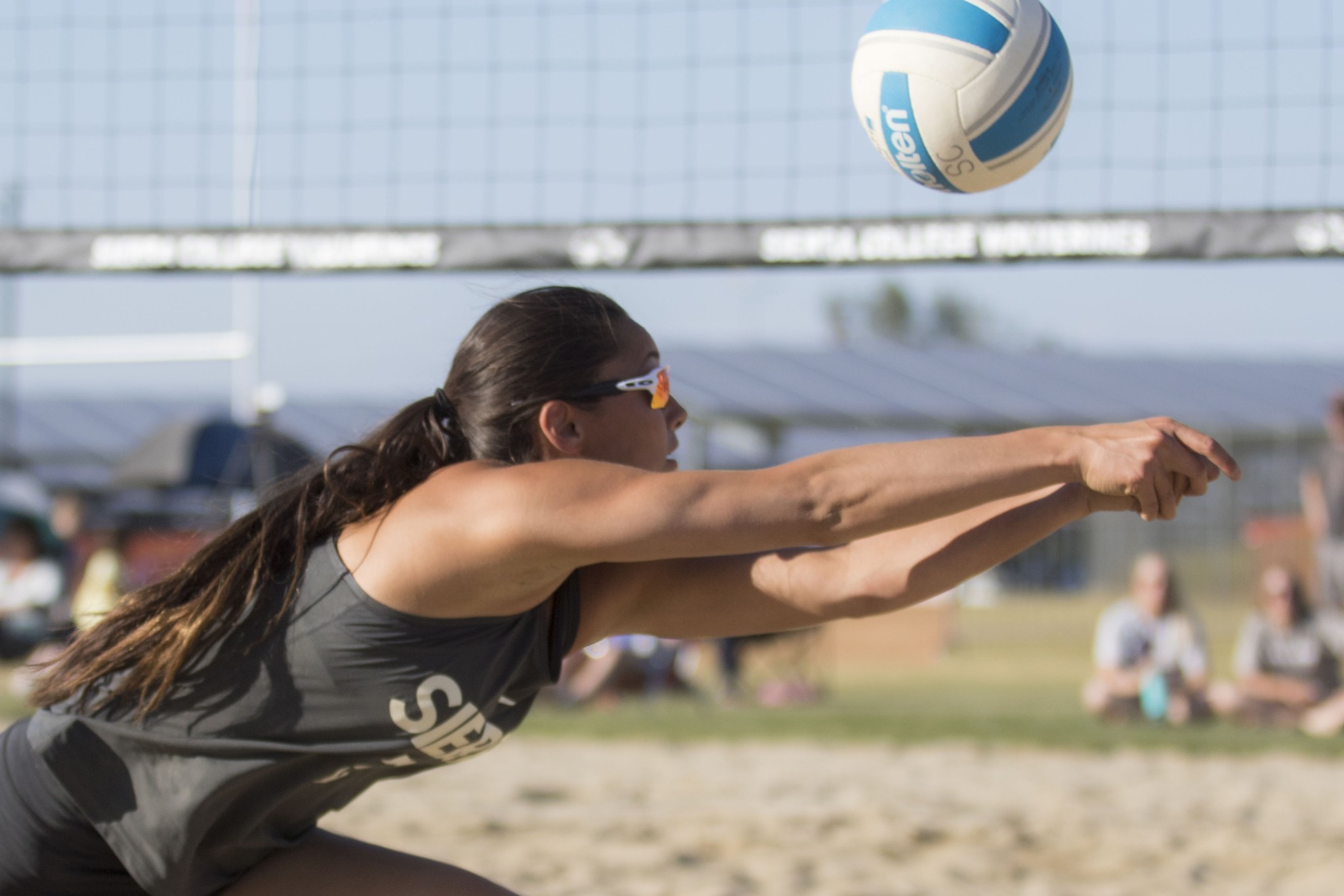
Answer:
[512,364,672,411]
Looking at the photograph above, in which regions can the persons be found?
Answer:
[1206,565,1344,738]
[70,510,145,636]
[0,519,67,666]
[1083,552,1209,726]
[0,286,1238,895]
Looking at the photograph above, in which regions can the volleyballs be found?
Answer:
[851,0,1074,194]
